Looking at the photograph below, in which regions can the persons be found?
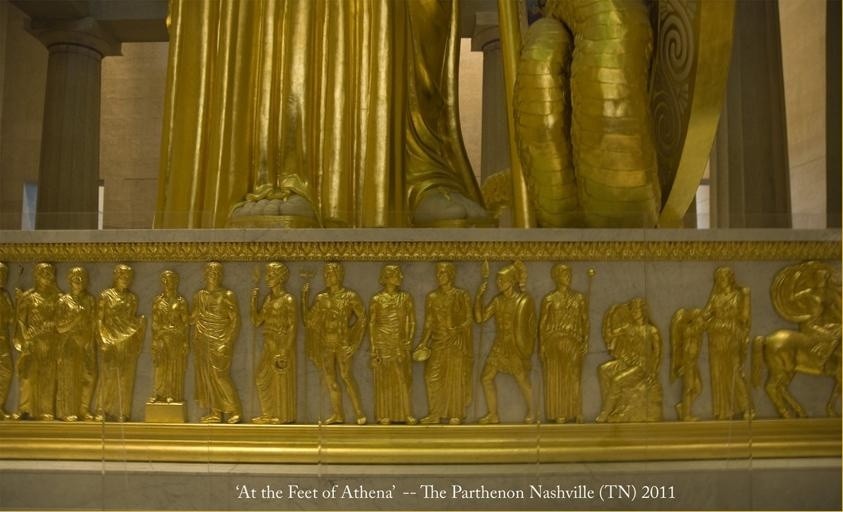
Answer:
[0,257,843,425]
[156,0,502,231]
